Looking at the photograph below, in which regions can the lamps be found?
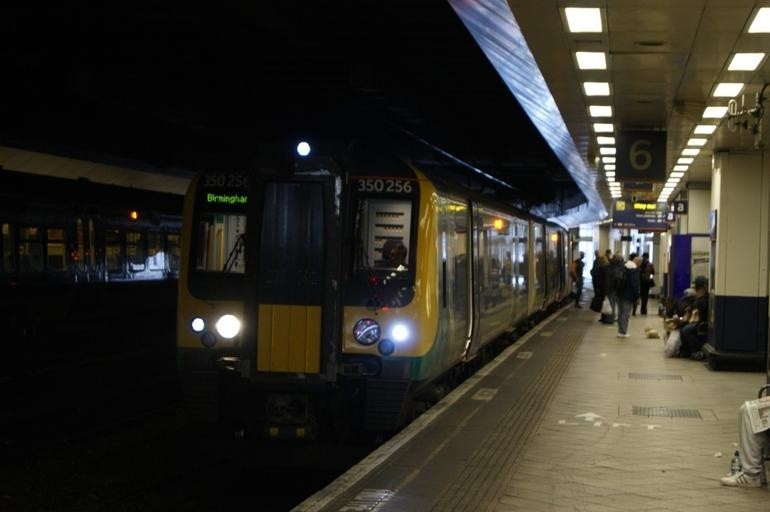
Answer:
[723,81,770,136]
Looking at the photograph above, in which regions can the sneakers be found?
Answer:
[617,333,631,338]
[721,468,764,488]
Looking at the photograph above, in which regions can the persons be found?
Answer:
[442,244,713,360]
[718,394,769,489]
[379,237,407,268]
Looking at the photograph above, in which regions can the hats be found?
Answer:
[679,288,696,301]
[623,260,637,269]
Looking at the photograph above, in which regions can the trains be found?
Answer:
[177,134,574,444]
[0,133,201,358]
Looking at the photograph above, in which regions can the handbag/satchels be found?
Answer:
[664,327,682,359]
